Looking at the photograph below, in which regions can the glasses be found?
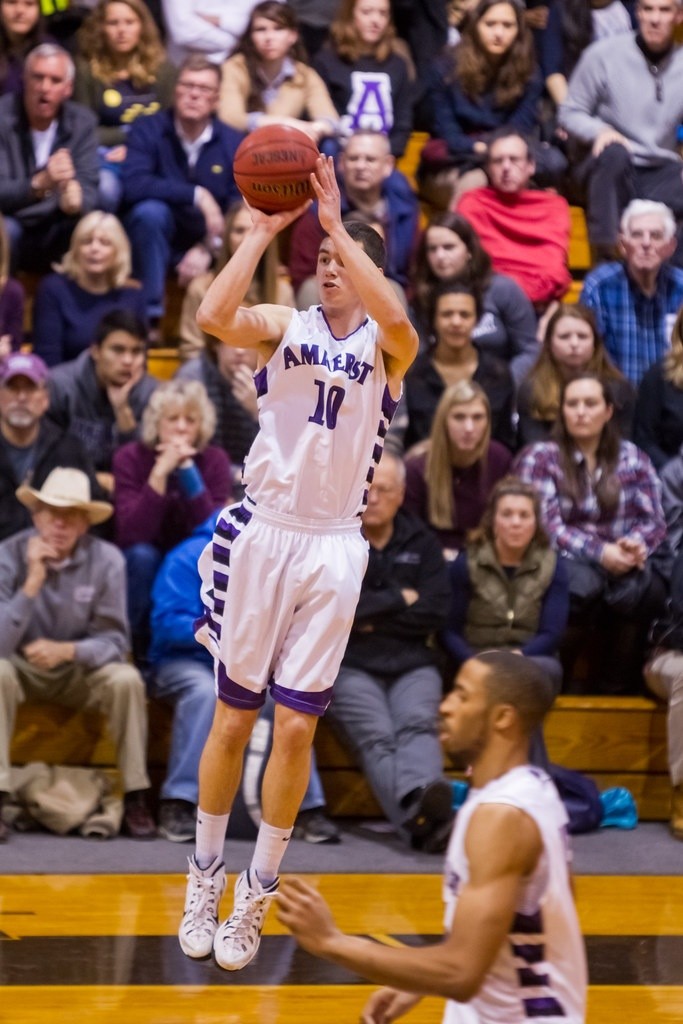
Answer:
[177,80,218,92]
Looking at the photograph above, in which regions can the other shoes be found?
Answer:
[411,781,454,854]
[124,797,155,835]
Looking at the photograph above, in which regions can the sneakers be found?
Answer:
[178,854,227,958]
[293,813,338,843]
[157,804,196,842]
[213,867,280,971]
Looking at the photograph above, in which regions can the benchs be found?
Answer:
[144,130,597,383]
[11,686,674,826]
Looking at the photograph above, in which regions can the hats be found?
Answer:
[15,466,113,525]
[0,353,49,389]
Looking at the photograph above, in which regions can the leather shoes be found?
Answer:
[668,796,683,839]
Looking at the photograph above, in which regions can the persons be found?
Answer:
[271,651,588,1024]
[180,153,418,971]
[0,0,682,853]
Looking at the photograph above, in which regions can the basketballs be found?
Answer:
[233,124,321,213]
